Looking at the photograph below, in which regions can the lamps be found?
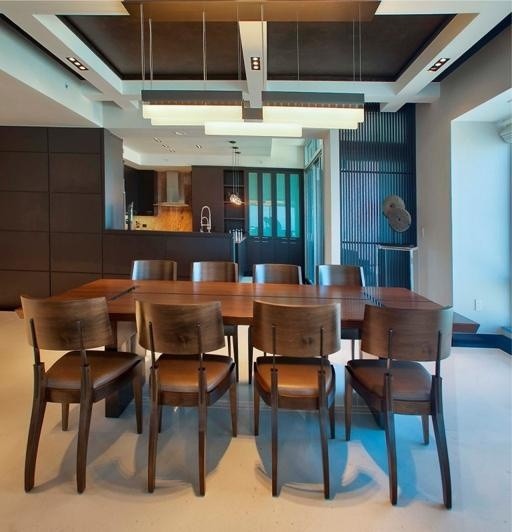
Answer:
[138,4,364,137]
[227,140,243,206]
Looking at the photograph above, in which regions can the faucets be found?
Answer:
[200,205,212,233]
[200,217,208,232]
